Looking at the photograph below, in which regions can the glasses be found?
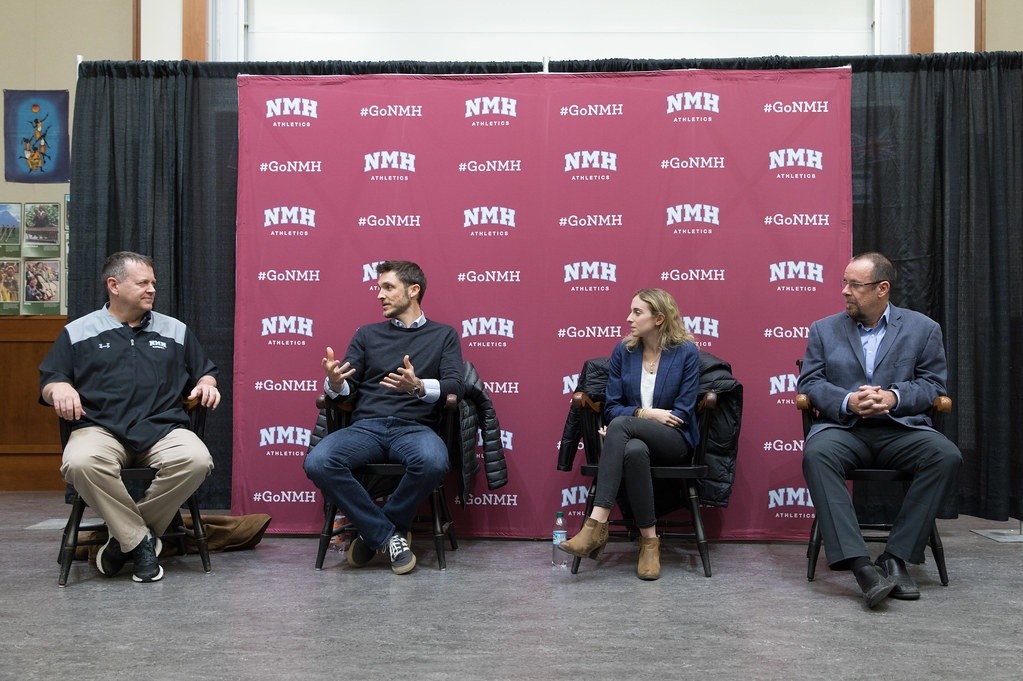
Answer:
[840,279,888,292]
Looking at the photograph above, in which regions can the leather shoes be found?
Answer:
[874,554,921,601]
[852,565,897,607]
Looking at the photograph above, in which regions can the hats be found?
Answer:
[5,265,16,271]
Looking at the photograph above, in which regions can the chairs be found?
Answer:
[794,359,952,587]
[571,358,714,579]
[58,396,211,587]
[315,360,475,573]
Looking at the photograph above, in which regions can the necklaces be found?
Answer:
[644,349,661,374]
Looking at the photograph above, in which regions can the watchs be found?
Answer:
[406,378,421,396]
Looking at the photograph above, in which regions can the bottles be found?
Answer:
[552,512,567,568]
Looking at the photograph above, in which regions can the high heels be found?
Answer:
[558,517,609,560]
[637,534,662,581]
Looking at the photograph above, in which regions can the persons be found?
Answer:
[38,251,222,583]
[557,288,700,581]
[304,260,466,574]
[797,252,964,609]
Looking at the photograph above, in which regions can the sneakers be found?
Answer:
[133,526,164,583]
[382,531,416,574]
[347,535,379,568]
[96,524,126,576]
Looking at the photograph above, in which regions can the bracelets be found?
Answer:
[638,409,648,418]
